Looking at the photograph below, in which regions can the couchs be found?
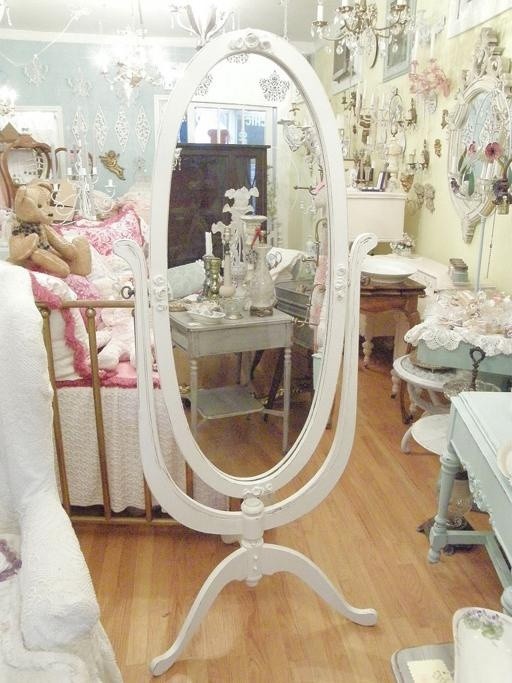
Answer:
[1,190,231,531]
[168,246,327,304]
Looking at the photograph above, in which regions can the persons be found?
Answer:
[101,151,125,180]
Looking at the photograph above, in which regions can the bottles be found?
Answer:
[448,257,469,283]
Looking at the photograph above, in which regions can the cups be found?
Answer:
[220,296,246,321]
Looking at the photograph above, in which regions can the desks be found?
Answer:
[426,391,511,610]
[395,323,511,423]
[360,271,427,426]
[240,278,323,421]
[341,189,406,240]
[168,297,296,457]
[392,347,511,473]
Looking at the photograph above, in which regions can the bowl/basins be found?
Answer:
[360,256,417,283]
[185,307,225,325]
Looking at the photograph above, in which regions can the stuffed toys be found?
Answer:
[10,181,91,277]
[94,298,155,371]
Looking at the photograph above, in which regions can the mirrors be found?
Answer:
[440,24,512,242]
[114,26,377,678]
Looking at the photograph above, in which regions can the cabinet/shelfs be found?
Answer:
[227,144,271,242]
[167,142,227,267]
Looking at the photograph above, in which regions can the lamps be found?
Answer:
[311,0,410,66]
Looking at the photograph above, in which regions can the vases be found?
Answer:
[247,244,277,308]
[237,214,268,309]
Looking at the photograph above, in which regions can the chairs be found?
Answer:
[1,257,124,681]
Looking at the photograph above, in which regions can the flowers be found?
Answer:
[449,140,477,196]
[483,141,512,213]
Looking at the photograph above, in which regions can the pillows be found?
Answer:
[15,204,146,389]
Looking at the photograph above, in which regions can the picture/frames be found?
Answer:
[332,28,352,81]
[383,0,417,83]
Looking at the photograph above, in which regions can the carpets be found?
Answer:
[390,642,456,683]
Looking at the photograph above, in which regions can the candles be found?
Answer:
[410,20,421,62]
[336,81,386,130]
[52,164,114,192]
[428,14,435,61]
[205,229,213,258]
[221,251,232,289]
